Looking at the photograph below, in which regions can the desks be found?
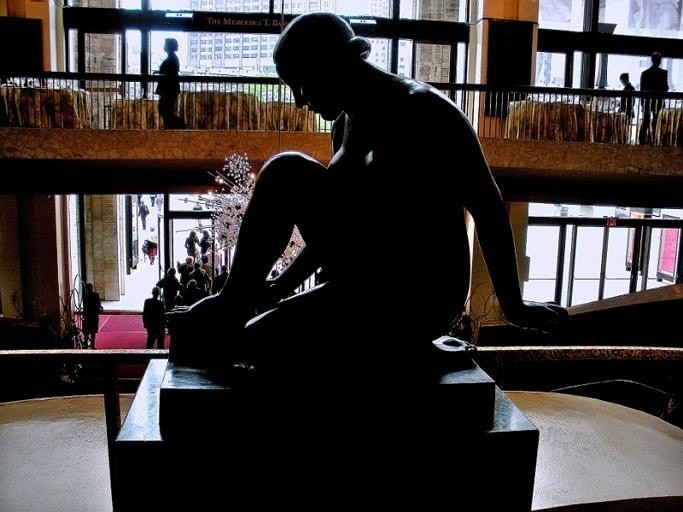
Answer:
[504,100,683,146]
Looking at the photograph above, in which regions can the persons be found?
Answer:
[149,194,157,207]
[615,72,634,141]
[136,201,149,229]
[160,14,570,383]
[141,229,227,350]
[136,194,142,204]
[81,281,102,350]
[638,50,668,144]
[153,36,182,120]
